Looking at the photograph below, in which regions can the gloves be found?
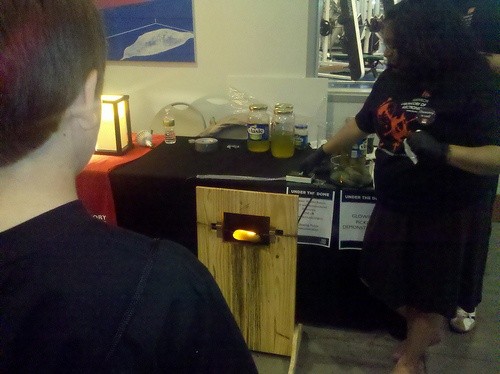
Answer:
[299,144,329,176]
[406,129,445,155]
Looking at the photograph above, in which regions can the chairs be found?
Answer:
[150,101,206,137]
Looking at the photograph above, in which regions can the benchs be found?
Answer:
[334,54,384,78]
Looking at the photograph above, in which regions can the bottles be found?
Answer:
[163,104,177,144]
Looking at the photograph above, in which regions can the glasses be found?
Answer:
[378,43,397,53]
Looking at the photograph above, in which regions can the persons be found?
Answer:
[0,0,258,374]
[299,0,500,374]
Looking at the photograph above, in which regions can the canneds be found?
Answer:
[246,103,270,151]
[271,103,295,158]
[294,124,308,150]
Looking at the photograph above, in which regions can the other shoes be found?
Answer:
[449,305,477,334]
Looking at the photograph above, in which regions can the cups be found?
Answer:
[331,156,349,184]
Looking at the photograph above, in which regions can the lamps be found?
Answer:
[94,94,133,156]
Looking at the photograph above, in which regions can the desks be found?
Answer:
[75,131,408,340]
